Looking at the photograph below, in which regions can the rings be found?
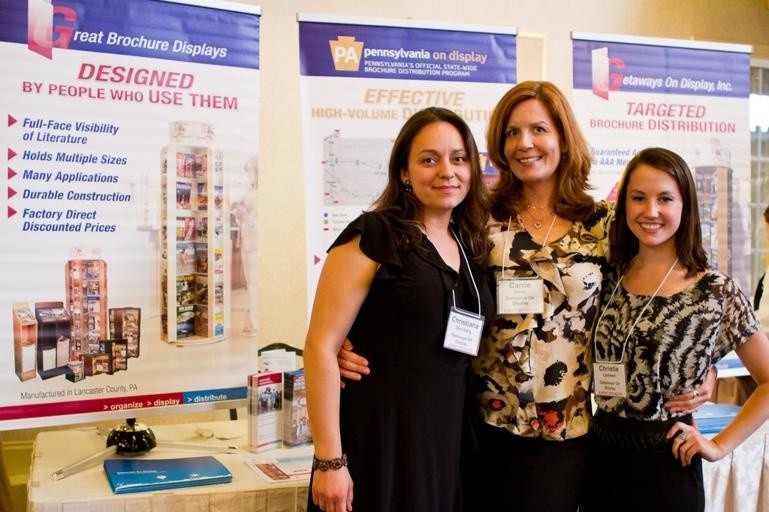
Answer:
[678,432,687,442]
[693,391,699,399]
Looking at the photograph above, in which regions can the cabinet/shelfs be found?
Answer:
[6,255,142,386]
[154,140,254,349]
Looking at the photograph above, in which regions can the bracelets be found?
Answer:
[312,453,349,472]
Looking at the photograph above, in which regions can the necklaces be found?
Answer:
[526,205,554,230]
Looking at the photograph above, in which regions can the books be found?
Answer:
[103,456,233,495]
[246,370,313,457]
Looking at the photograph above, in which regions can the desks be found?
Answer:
[688,398,768,512]
[25,401,315,512]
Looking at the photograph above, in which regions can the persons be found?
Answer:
[338,80,718,512]
[303,108,498,512]
[575,147,769,512]
[735,206,769,406]
